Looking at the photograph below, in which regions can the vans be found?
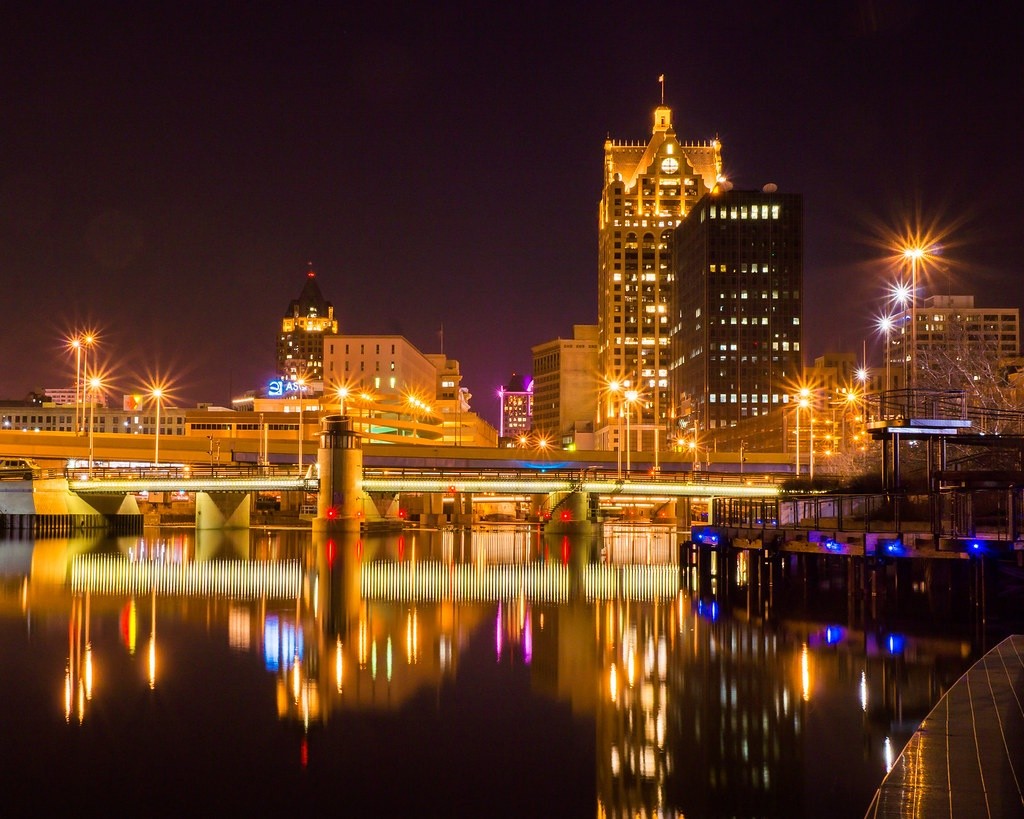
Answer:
[0,458,41,480]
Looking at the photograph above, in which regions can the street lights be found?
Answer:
[152,387,164,464]
[89,376,102,480]
[625,391,639,477]
[71,340,80,436]
[406,395,432,438]
[81,335,94,435]
[903,246,924,417]
[878,318,893,415]
[360,392,376,443]
[297,377,306,476]
[893,285,910,414]
[608,380,624,483]
[336,387,349,415]
[799,388,814,476]
[796,400,809,478]
